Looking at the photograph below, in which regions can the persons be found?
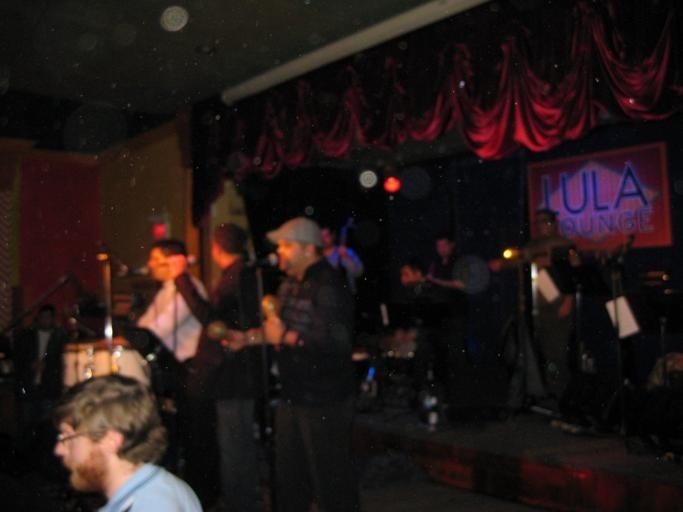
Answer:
[608,233,642,272]
[516,207,586,432]
[219,215,359,511]
[202,223,265,511]
[51,372,203,512]
[132,240,211,452]
[401,234,479,423]
[321,227,363,298]
[13,303,66,454]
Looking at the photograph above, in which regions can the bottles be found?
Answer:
[417,369,442,429]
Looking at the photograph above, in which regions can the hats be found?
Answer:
[264,216,326,249]
[211,222,247,254]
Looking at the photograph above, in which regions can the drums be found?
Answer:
[61,342,103,388]
[93,338,151,387]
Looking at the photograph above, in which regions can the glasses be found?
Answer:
[45,430,94,453]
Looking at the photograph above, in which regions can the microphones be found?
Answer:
[97,240,128,278]
[158,255,197,267]
[617,234,635,263]
[244,253,279,268]
